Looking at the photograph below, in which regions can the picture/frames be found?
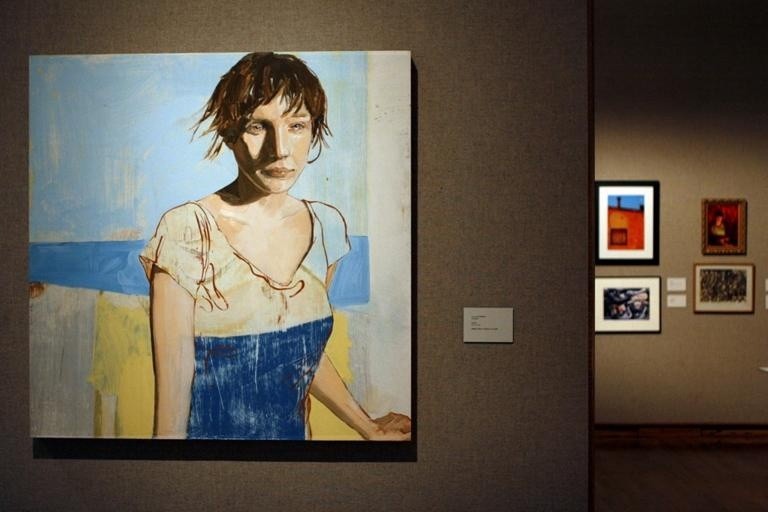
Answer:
[595,180,660,266]
[594,277,662,334]
[693,263,756,314]
[700,196,747,256]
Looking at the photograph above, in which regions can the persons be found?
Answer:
[710,209,735,247]
[136,52,412,440]
